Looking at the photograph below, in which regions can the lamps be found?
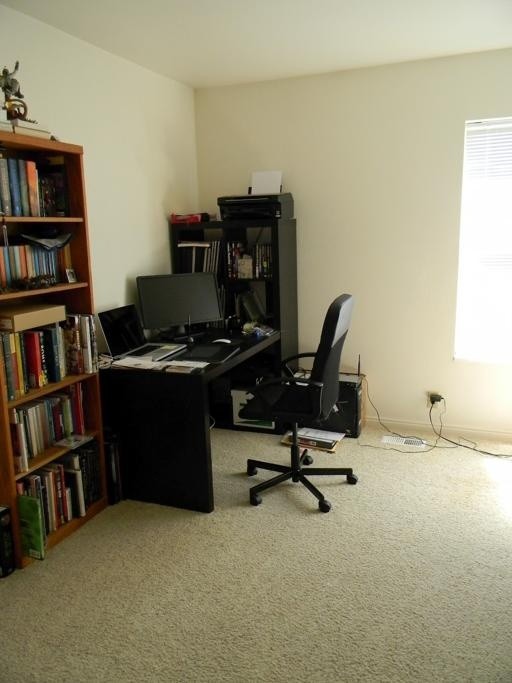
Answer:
[172,238,210,271]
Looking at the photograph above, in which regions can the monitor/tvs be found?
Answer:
[136,272,225,341]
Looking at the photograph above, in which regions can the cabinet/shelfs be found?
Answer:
[0,127,108,579]
[169,217,300,385]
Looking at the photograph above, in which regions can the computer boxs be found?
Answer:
[287,370,367,439]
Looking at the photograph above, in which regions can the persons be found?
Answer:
[0,60,24,100]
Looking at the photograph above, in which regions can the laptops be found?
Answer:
[97,304,188,361]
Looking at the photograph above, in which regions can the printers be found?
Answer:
[217,186,294,222]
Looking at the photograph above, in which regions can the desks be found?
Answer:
[99,324,279,513]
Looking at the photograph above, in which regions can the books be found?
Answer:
[203,241,274,320]
[281,427,346,452]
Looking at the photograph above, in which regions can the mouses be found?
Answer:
[209,336,233,344]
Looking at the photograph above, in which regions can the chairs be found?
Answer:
[237,290,361,516]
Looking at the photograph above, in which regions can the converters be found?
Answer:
[430,395,441,402]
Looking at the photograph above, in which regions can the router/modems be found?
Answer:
[338,353,364,388]
